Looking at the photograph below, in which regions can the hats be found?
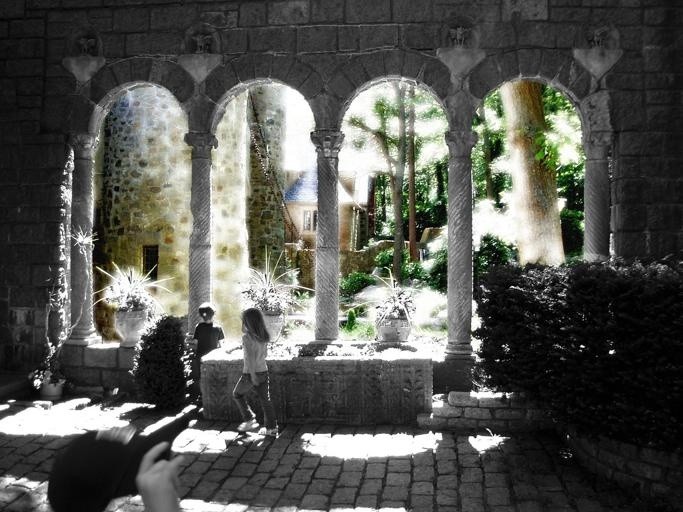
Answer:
[199,303,217,316]
[48,429,170,511]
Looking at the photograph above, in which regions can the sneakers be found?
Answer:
[259,427,277,436]
[237,418,260,432]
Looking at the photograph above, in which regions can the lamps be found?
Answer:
[176,33,223,85]
[573,28,624,80]
[436,27,486,83]
[62,35,106,83]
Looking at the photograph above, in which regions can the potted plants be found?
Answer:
[86,262,176,348]
[28,224,99,401]
[342,267,420,344]
[241,244,315,344]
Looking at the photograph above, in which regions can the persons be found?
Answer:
[232,308,279,436]
[134,440,187,512]
[192,301,224,403]
